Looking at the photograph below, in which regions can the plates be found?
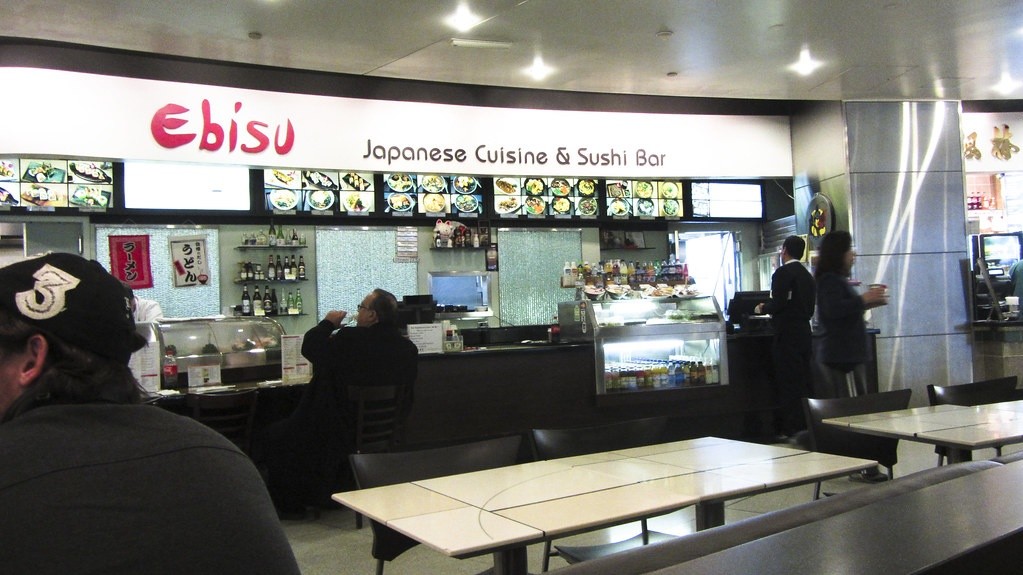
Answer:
[264,169,372,213]
[645,287,670,299]
[386,174,478,213]
[606,179,680,217]
[493,178,598,215]
[0,159,110,207]
[674,285,699,298]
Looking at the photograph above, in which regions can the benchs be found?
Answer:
[543,453,1007,575]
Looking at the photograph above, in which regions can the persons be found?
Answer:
[0,253,300,575]
[813,230,887,400]
[1009,259,1023,313]
[758,235,813,447]
[119,281,163,343]
[269,287,418,521]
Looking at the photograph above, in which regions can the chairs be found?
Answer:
[350,415,677,575]
[318,384,400,530]
[801,376,1023,501]
[191,387,259,466]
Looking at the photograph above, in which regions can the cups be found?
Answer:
[869,284,887,291]
[341,312,357,326]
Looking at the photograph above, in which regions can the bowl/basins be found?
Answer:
[605,285,630,300]
[583,287,606,300]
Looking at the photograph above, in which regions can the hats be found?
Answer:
[0,253,135,366]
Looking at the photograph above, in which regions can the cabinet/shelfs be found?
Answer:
[230,245,305,315]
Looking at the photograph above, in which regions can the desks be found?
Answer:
[821,399,1023,467]
[329,433,879,575]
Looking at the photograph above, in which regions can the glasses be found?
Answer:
[357,305,369,314]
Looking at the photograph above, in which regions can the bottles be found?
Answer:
[603,355,720,392]
[551,316,559,343]
[240,218,306,246]
[238,255,305,281]
[563,259,689,286]
[242,284,303,315]
[163,351,178,391]
[435,227,480,248]
